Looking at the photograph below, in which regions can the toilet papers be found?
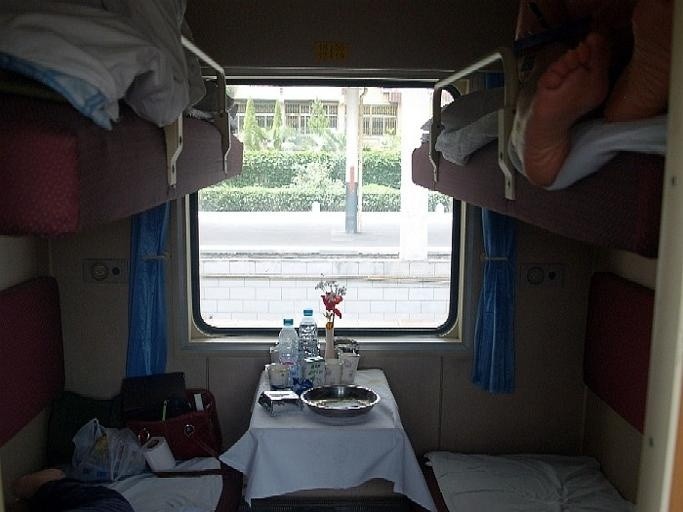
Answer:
[144,437,176,472]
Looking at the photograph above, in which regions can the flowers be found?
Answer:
[315,272,348,322]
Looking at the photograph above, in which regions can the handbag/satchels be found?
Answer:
[45,392,120,466]
[126,411,222,460]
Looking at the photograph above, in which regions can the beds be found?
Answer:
[425,448,635,512]
[410,47,661,290]
[35,430,243,511]
[0,30,245,240]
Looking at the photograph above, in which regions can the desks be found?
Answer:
[249,368,402,445]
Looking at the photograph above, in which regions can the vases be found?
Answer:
[324,325,336,359]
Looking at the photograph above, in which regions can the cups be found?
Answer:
[266,354,360,389]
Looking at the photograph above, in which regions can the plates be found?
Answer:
[299,385,382,419]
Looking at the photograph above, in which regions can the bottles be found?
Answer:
[278,309,320,375]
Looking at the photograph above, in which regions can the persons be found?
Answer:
[12,468,135,512]
[512,1,674,189]
